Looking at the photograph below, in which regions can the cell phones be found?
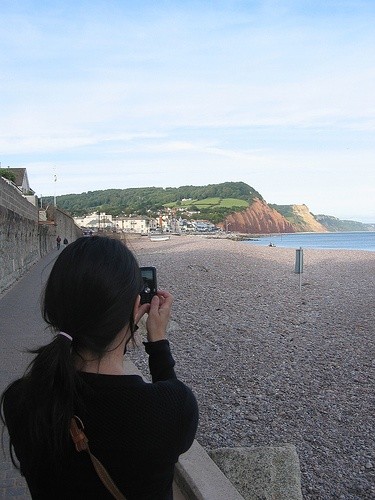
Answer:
[140,267,157,306]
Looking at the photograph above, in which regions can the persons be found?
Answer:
[1,233,201,499]
[55,234,62,251]
[63,237,69,248]
